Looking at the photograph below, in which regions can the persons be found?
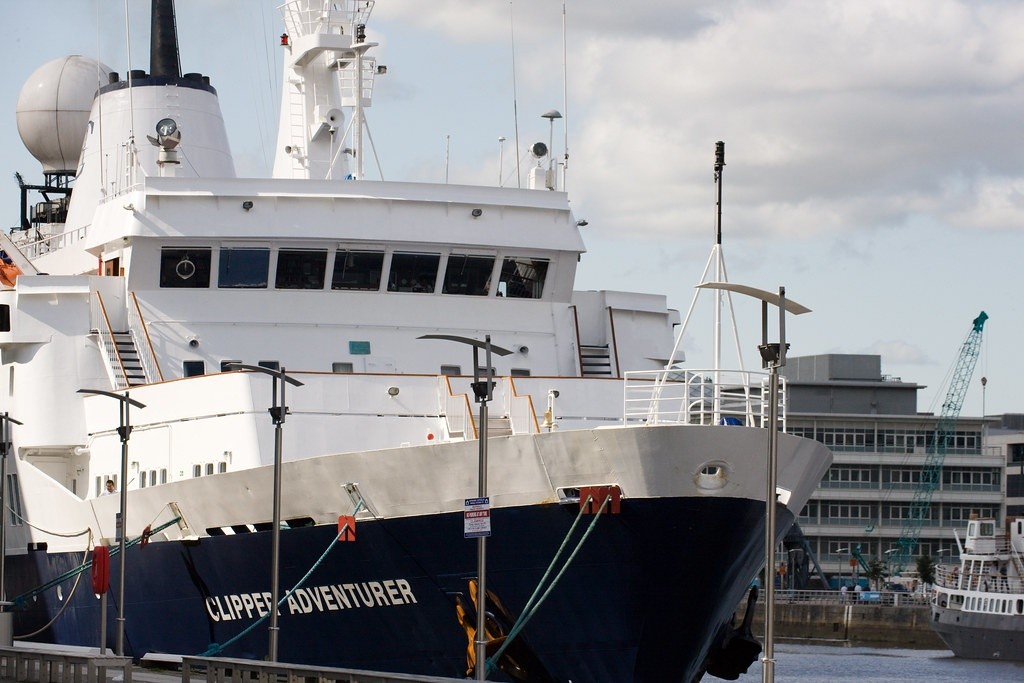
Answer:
[948,559,1010,594]
[751,581,875,604]
[413,273,434,293]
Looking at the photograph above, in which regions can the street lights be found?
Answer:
[415,333,514,681]
[72,385,145,656]
[222,361,304,661]
[836,543,851,594]
[884,547,899,589]
[696,282,814,683]
[0,410,23,610]
[936,548,950,563]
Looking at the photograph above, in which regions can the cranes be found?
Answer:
[849,309,989,591]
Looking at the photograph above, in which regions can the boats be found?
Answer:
[928,518,1023,660]
[1,0,834,683]
